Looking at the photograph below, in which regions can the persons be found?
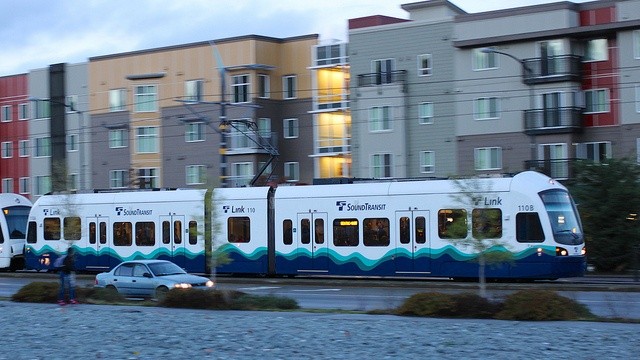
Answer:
[54,247,79,306]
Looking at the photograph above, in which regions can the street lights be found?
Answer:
[483,49,537,170]
[29,98,82,194]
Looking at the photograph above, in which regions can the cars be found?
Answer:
[94,259,215,302]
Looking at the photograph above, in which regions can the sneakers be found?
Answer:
[70,299,78,304]
[58,300,67,305]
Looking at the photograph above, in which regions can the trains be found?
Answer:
[0,171,587,281]
